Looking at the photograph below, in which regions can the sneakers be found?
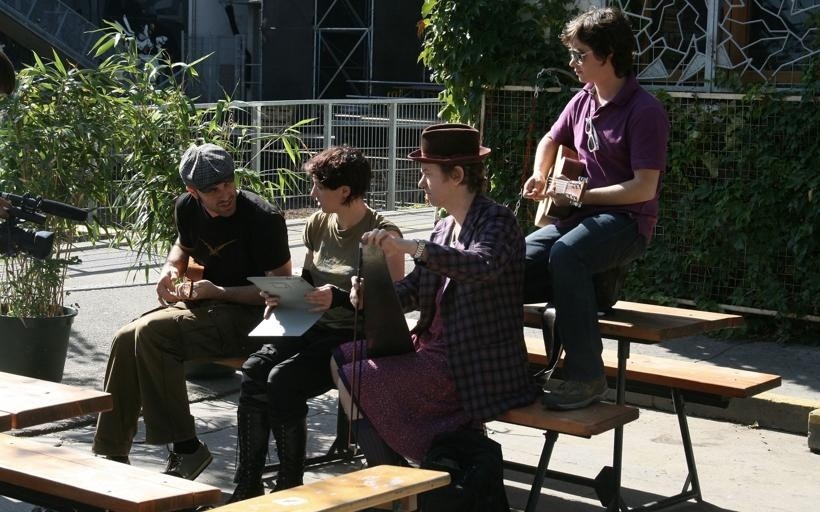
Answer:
[540,374,609,410]
[160,439,214,481]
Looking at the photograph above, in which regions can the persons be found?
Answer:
[521,6,674,410]
[219,143,405,506]
[326,123,528,511]
[92,141,291,481]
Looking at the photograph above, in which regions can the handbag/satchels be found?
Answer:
[415,428,511,512]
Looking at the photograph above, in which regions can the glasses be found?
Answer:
[584,117,600,153]
[568,48,593,66]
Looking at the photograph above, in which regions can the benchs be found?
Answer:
[0,431,220,512]
[206,464,452,512]
[525,339,781,512]
[210,354,639,512]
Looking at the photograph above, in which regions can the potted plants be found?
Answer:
[0,51,118,383]
[81,33,319,386]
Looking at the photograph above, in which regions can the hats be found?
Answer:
[407,123,492,164]
[178,142,234,190]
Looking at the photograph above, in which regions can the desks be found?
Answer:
[0,370,114,434]
[521,299,742,512]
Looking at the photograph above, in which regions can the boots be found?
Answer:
[225,410,271,505]
[270,418,307,494]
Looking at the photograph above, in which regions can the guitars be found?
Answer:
[534,144,588,227]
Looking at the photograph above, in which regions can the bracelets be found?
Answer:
[410,237,425,260]
